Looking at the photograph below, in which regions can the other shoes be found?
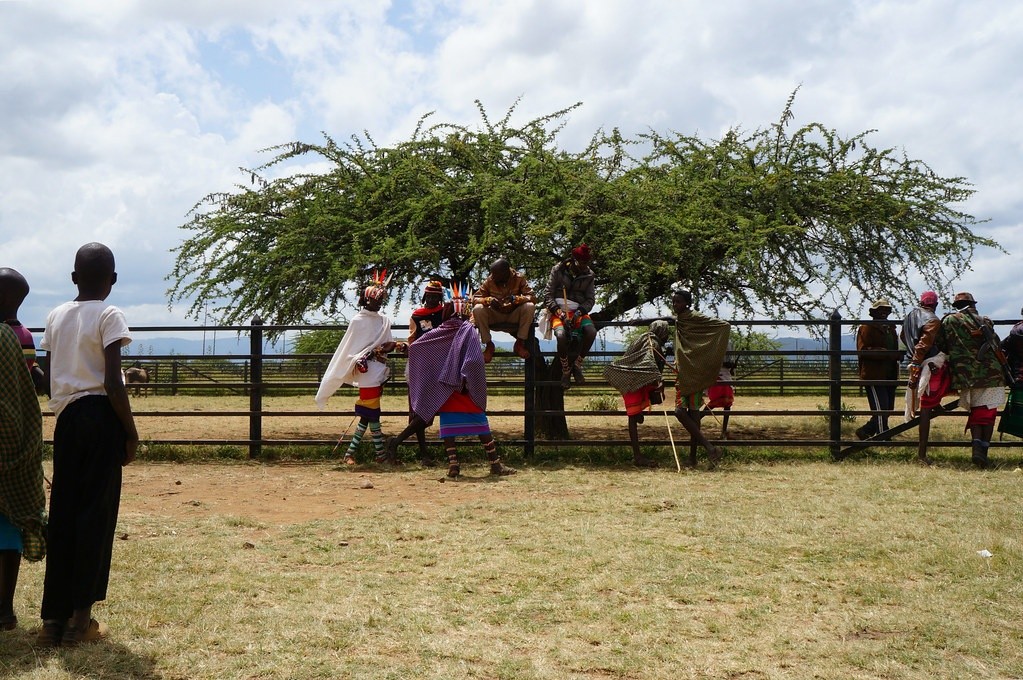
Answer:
[561,374,571,389]
[490,464,517,477]
[342,456,358,466]
[63,619,111,646]
[572,371,584,385]
[386,437,397,464]
[373,457,385,463]
[634,460,658,467]
[708,447,723,472]
[35,622,61,646]
[514,343,530,359]
[446,466,459,477]
[482,345,495,363]
[420,458,431,466]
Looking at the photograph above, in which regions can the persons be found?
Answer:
[997,308,1023,441]
[0,267,48,631]
[37,242,138,646]
[603,320,675,467]
[900,291,950,457]
[315,287,395,465]
[700,341,738,440]
[536,244,597,388]
[930,293,1005,469]
[470,259,536,363]
[408,303,516,477]
[854,300,904,441]
[380,279,445,466]
[672,291,731,469]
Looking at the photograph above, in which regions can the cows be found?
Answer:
[125,367,152,398]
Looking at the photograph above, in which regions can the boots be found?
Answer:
[971,440,988,467]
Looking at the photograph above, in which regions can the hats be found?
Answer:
[921,292,937,304]
[869,298,892,315]
[571,243,592,261]
[422,280,443,304]
[952,292,977,306]
